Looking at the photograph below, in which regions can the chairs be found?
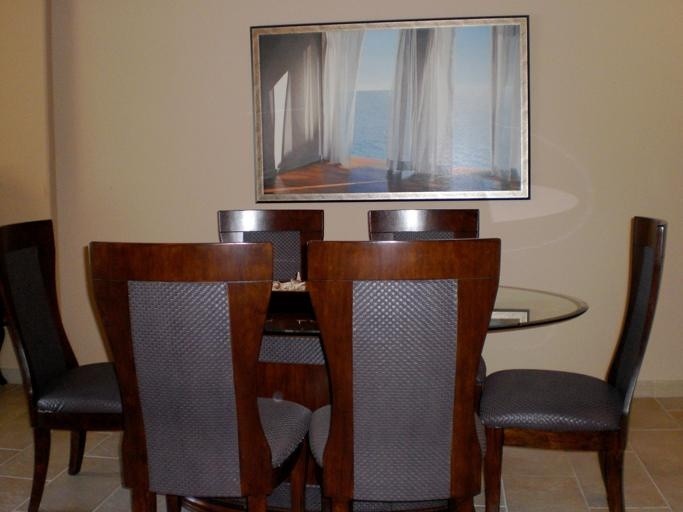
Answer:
[0,213,668,511]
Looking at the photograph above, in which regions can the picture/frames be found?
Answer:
[250,15,530,211]
